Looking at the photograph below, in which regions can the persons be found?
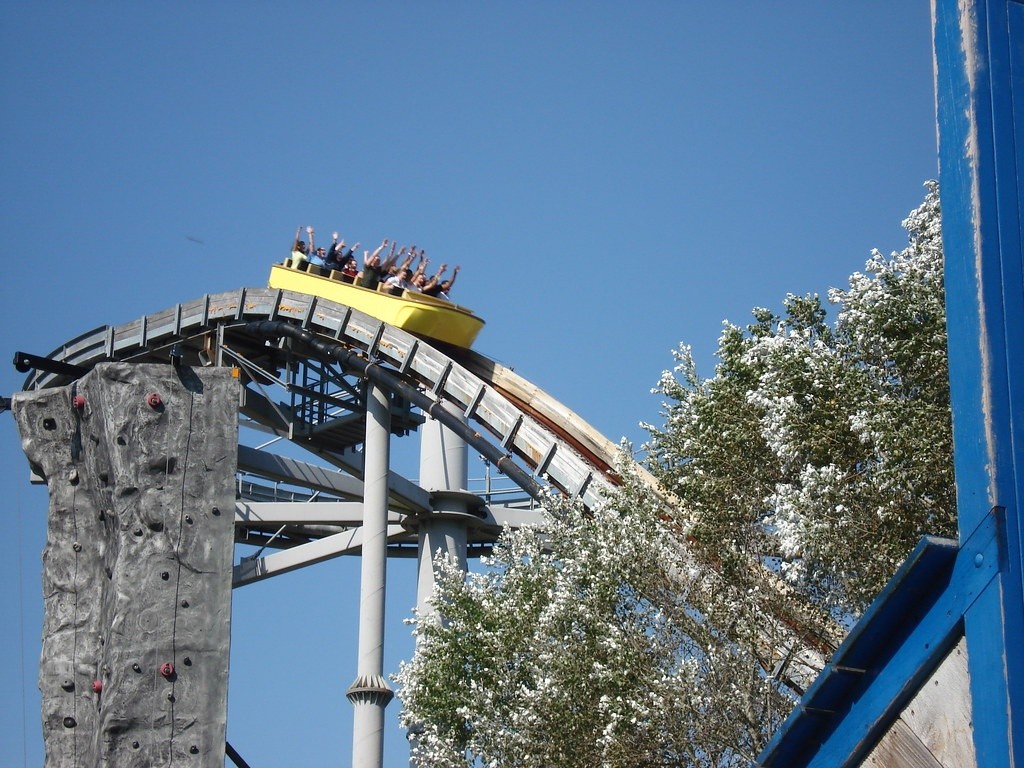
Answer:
[289,225,461,297]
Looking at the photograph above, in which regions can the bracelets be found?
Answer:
[398,252,401,256]
[390,249,394,253]
[408,252,411,255]
[379,247,382,250]
